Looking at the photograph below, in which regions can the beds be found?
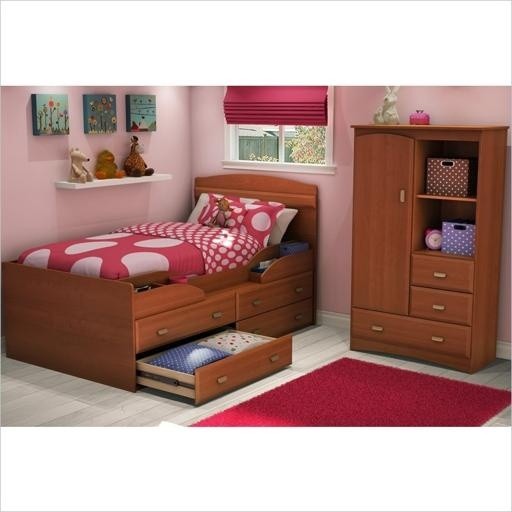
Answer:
[0,172,320,407]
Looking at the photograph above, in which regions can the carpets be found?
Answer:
[185,356,511,426]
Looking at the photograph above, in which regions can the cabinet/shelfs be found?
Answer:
[349,120,510,375]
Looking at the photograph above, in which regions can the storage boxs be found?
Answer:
[440,221,475,258]
[425,156,470,198]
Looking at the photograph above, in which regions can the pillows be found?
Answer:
[185,191,299,249]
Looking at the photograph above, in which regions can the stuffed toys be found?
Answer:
[67,146,95,184]
[122,135,156,180]
[94,150,127,180]
[202,197,233,229]
[372,86,402,125]
